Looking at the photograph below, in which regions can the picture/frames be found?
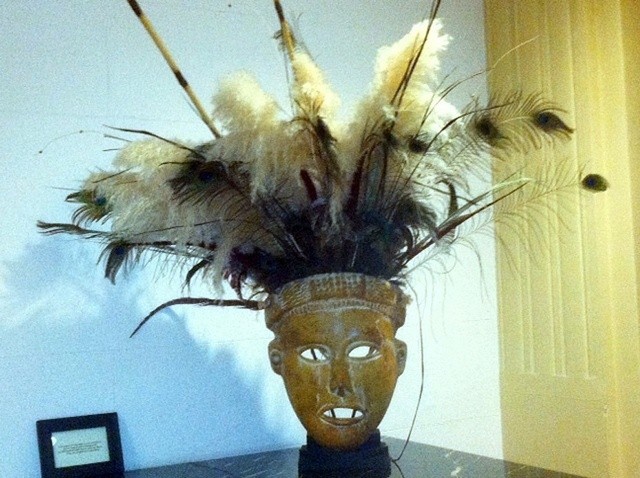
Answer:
[36,412,125,478]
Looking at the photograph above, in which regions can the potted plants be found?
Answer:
[32,2,611,469]
